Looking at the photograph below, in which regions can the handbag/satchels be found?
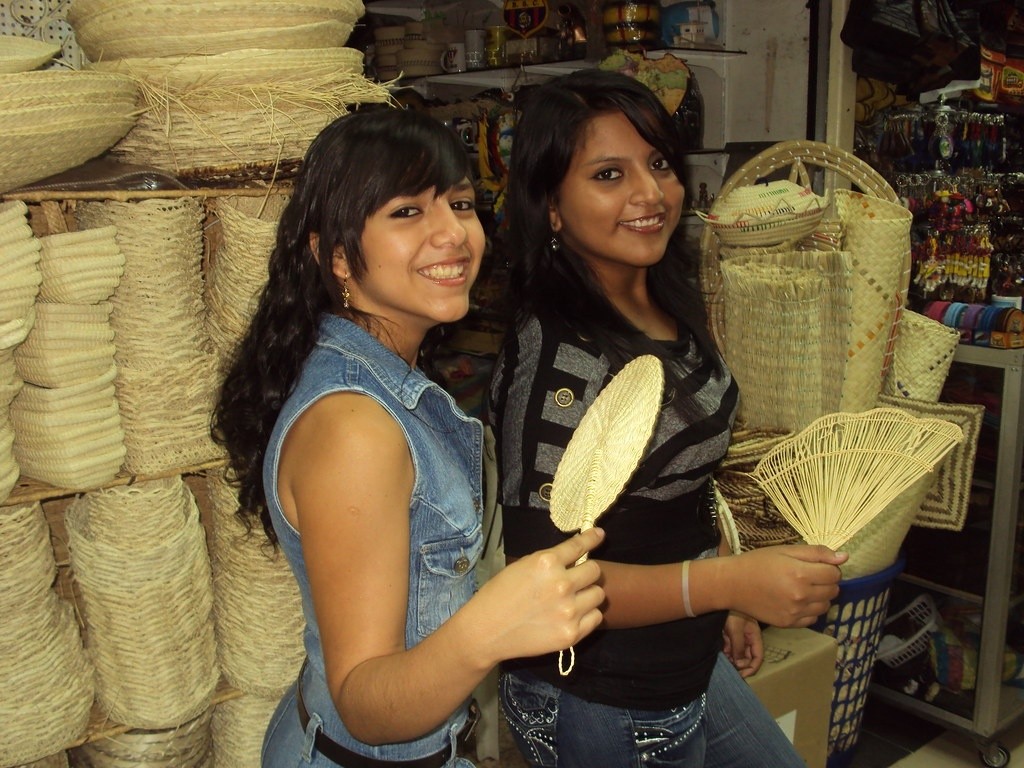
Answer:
[840,0,981,95]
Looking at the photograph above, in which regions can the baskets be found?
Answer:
[878,594,942,668]
[0,0,397,768]
[696,181,830,246]
[374,22,440,79]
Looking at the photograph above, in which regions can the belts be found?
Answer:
[296,656,482,768]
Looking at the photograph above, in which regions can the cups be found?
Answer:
[441,43,466,73]
[452,117,480,152]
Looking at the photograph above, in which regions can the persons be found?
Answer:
[483,67,848,767]
[210,110,606,767]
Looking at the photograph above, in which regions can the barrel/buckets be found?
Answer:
[603,0,660,52]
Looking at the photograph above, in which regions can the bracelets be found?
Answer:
[682,560,696,617]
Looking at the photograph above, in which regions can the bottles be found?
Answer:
[464,30,486,68]
[486,26,507,66]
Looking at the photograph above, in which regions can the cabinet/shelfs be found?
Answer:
[866,345,1024,768]
[428,51,731,207]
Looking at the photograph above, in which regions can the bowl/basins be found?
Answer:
[373,22,472,80]
